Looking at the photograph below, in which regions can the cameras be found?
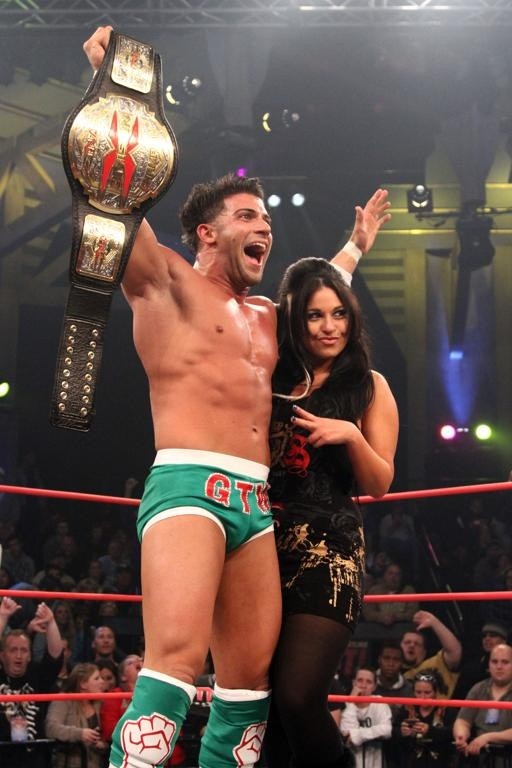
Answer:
[404,718,419,727]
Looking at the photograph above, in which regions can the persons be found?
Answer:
[268,256,401,767]
[83,27,393,768]
[1,501,512,766]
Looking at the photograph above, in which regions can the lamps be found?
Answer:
[259,108,300,133]
[166,75,203,105]
[406,185,434,214]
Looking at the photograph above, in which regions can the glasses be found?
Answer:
[481,631,500,638]
[416,672,434,680]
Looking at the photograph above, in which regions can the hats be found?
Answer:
[481,617,509,640]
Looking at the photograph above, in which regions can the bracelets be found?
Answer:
[340,241,363,264]
